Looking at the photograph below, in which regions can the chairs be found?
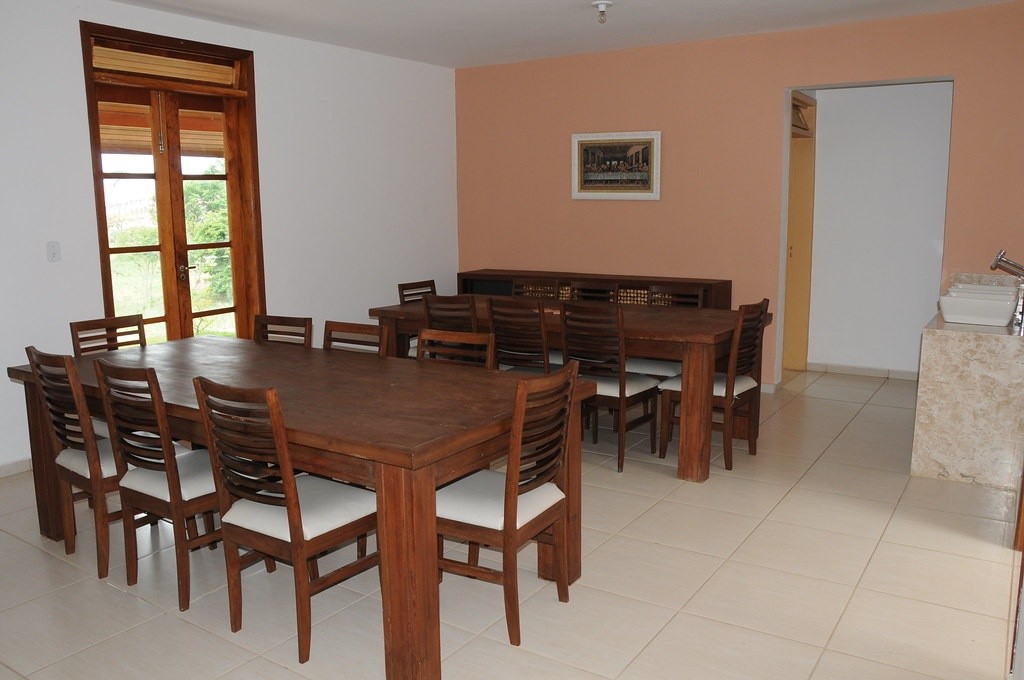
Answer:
[253,314,312,347]
[613,284,708,442]
[657,297,770,471]
[512,278,565,365]
[323,319,389,356]
[416,328,497,368]
[192,375,378,663]
[398,279,437,304]
[569,280,622,305]
[93,356,277,612]
[421,294,480,358]
[25,345,201,579]
[560,299,663,472]
[434,359,580,646]
[70,313,147,357]
[487,297,564,373]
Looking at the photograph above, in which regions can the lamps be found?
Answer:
[592,1,615,24]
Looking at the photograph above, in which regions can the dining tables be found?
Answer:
[369,294,773,484]
[6,334,597,680]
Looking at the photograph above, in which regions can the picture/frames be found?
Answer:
[571,130,660,201]
[792,107,809,130]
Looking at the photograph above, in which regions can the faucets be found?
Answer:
[991,248,1024,278]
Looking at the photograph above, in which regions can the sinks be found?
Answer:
[938,290,1019,331]
[946,282,1019,298]
